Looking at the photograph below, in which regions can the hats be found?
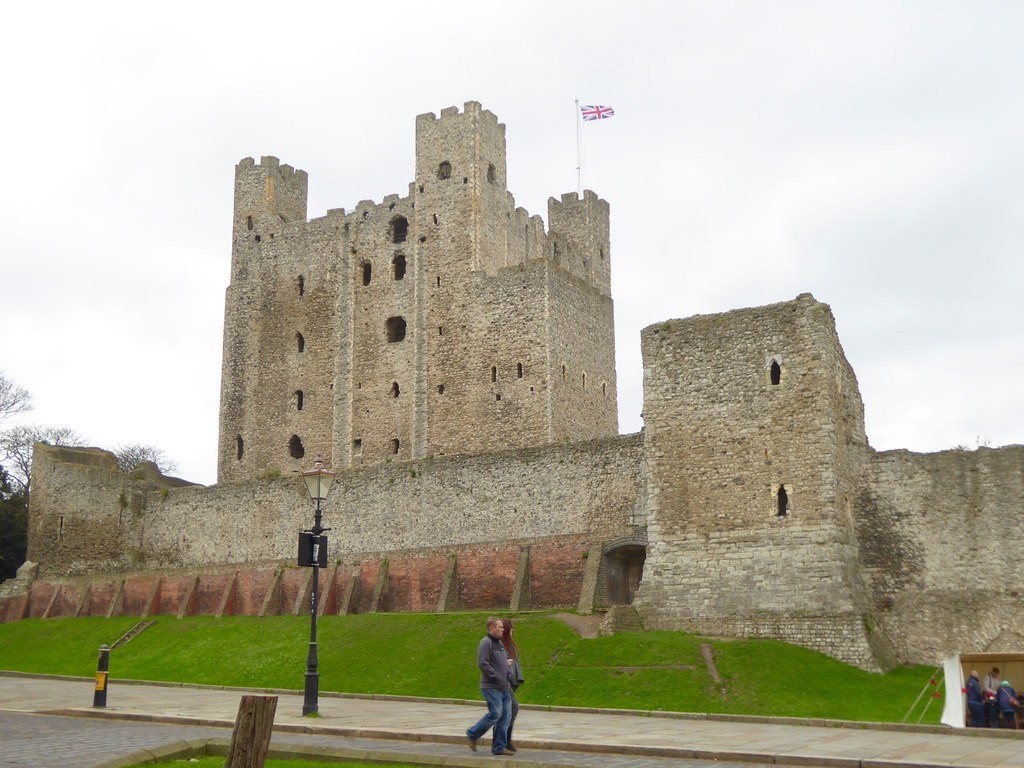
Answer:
[1001,682,1008,686]
[986,692,993,698]
[993,667,1000,673]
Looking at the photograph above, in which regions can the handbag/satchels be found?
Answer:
[1009,697,1020,708]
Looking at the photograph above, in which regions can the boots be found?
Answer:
[505,725,515,751]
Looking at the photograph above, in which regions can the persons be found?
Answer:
[966,667,1019,729]
[465,616,524,756]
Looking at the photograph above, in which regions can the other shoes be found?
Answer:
[467,735,477,752]
[495,748,513,755]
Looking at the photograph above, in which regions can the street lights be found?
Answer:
[295,452,337,718]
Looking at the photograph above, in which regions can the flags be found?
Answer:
[580,106,614,121]
